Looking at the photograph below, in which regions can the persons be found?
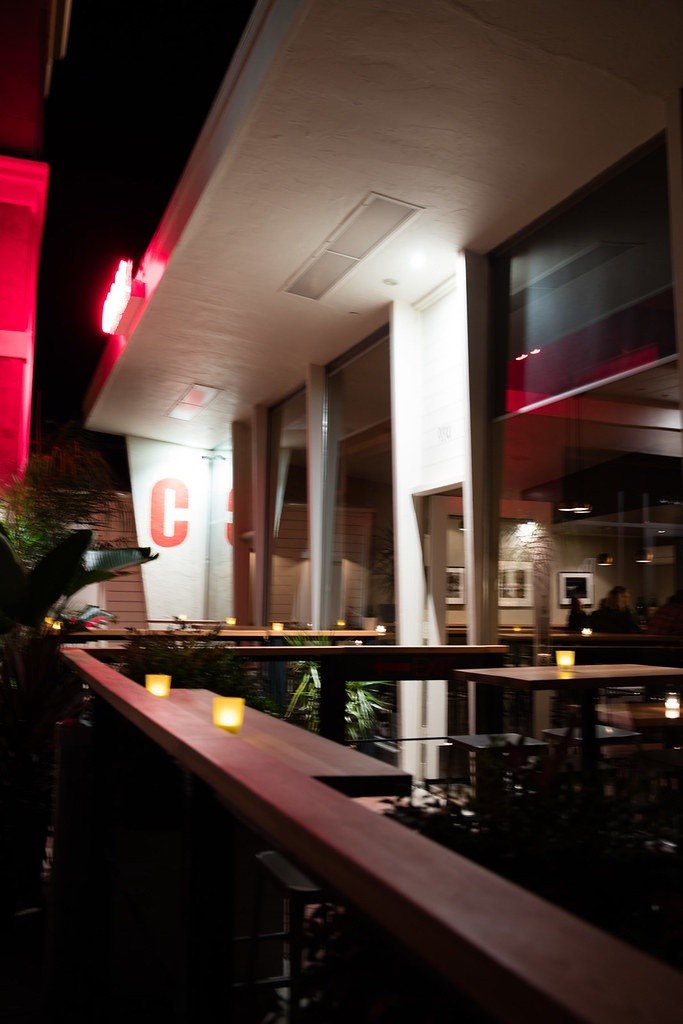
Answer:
[568,587,683,634]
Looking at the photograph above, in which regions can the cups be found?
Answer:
[225,617,237,625]
[144,674,172,697]
[211,697,245,733]
[555,650,575,669]
[336,620,346,626]
[271,622,284,631]
[664,691,681,717]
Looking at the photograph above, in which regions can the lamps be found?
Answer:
[596,552,613,566]
[634,548,655,563]
[556,370,593,514]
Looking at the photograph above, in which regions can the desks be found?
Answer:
[63,619,389,644]
[169,689,411,798]
[447,624,683,876]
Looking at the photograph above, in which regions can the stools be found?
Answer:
[447,733,550,862]
[541,725,643,845]
[638,747,682,855]
[257,851,336,1017]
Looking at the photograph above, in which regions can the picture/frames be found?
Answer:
[498,560,535,608]
[558,571,594,606]
[445,566,467,605]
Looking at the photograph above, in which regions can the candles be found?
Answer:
[212,697,246,737]
[556,650,575,670]
[226,618,237,626]
[272,623,284,632]
[145,674,172,698]
